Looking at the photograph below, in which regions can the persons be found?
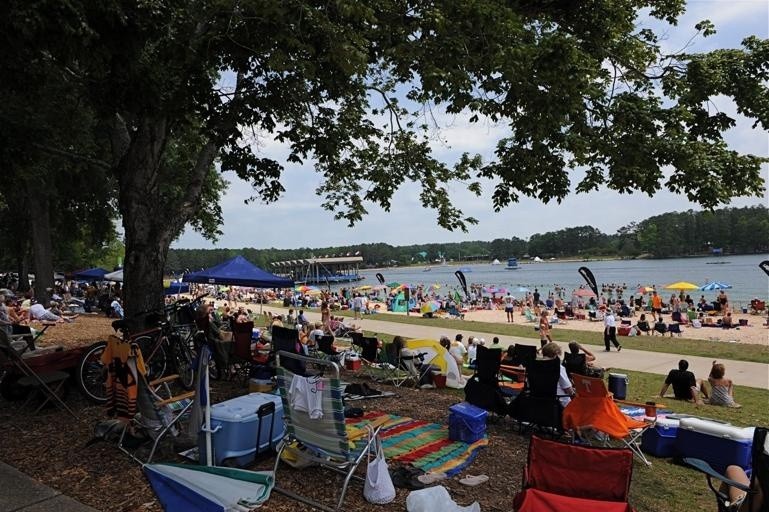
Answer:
[717,464,764,511]
[708,364,743,408]
[653,359,710,404]
[395,333,595,407]
[0,269,768,352]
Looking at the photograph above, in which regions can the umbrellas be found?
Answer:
[141,462,276,511]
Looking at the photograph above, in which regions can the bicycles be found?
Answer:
[166,292,222,381]
[78,296,195,405]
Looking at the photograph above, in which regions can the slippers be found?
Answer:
[417,472,448,484]
[459,474,489,486]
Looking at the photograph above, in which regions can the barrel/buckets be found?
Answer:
[344,351,361,370]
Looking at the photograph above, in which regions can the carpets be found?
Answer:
[344,408,480,482]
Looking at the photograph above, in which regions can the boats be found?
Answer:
[505,258,522,270]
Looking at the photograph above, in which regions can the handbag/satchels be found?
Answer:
[364,456,396,505]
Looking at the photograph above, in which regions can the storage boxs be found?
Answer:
[679,416,758,477]
[201,392,288,462]
[640,415,688,458]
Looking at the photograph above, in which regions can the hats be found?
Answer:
[604,308,613,314]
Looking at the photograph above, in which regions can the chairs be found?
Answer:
[1,278,124,357]
[681,425,766,511]
[163,279,767,389]
[523,357,571,437]
[236,350,386,510]
[568,372,660,469]
[102,333,201,469]
[468,343,524,417]
[2,327,78,422]
[516,435,633,510]
